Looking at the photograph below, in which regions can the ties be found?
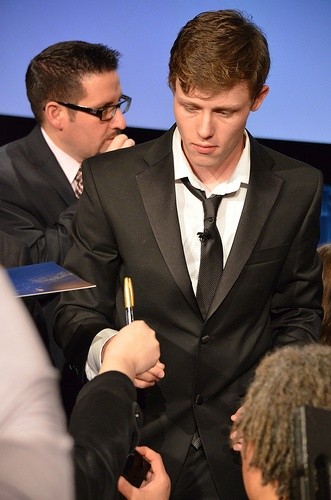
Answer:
[75,168,84,200]
[181,177,228,449]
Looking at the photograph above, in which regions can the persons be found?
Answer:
[0,241,331,499]
[0,39,136,340]
[50,9,325,500]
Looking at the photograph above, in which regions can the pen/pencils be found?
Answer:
[123,277,134,324]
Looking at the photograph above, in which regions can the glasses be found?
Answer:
[43,94,132,121]
